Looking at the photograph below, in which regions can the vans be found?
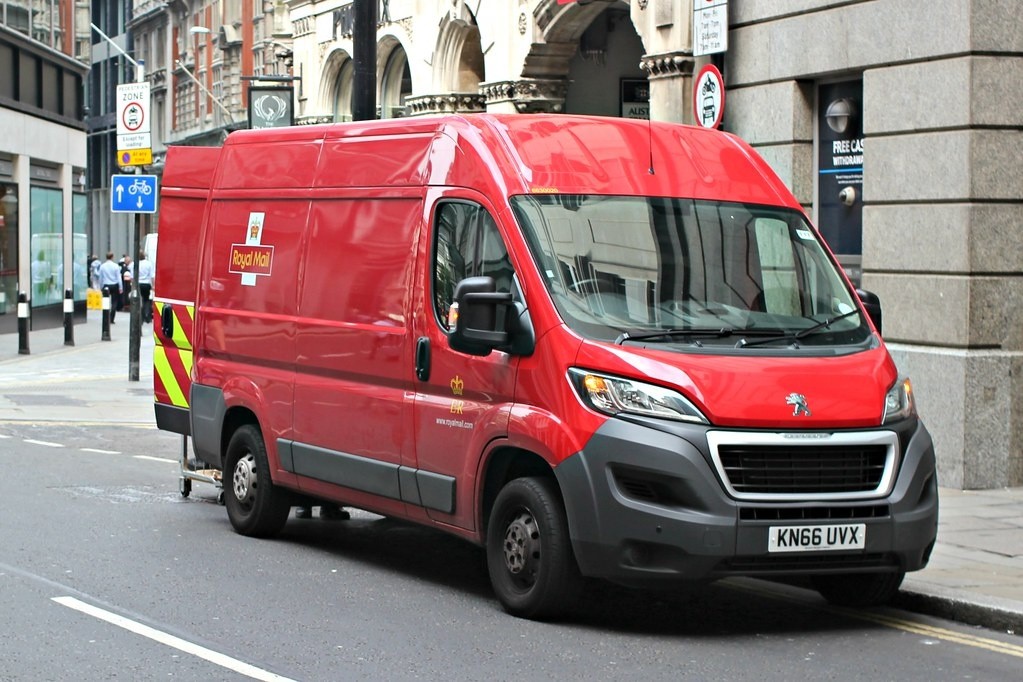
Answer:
[147,112,939,617]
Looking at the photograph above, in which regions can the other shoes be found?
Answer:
[295,507,312,518]
[320,506,350,521]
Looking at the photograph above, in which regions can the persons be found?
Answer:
[87,251,151,326]
[32,249,85,302]
